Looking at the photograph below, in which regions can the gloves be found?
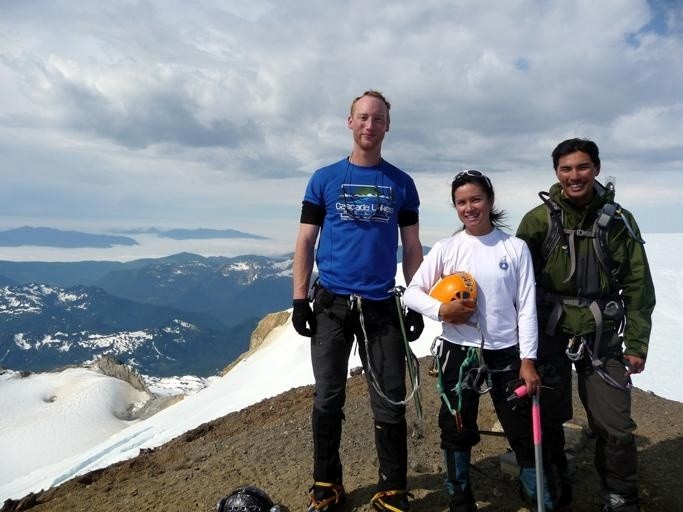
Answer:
[290,297,315,337]
[404,305,425,341]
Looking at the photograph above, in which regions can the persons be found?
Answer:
[292,89,424,512]
[513,138,656,512]
[401,168,560,510]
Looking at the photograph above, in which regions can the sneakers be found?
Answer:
[304,481,350,512]
[444,483,478,511]
[372,491,412,512]
[596,483,642,512]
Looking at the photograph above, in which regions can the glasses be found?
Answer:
[454,169,494,188]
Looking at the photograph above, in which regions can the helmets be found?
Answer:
[429,271,478,325]
